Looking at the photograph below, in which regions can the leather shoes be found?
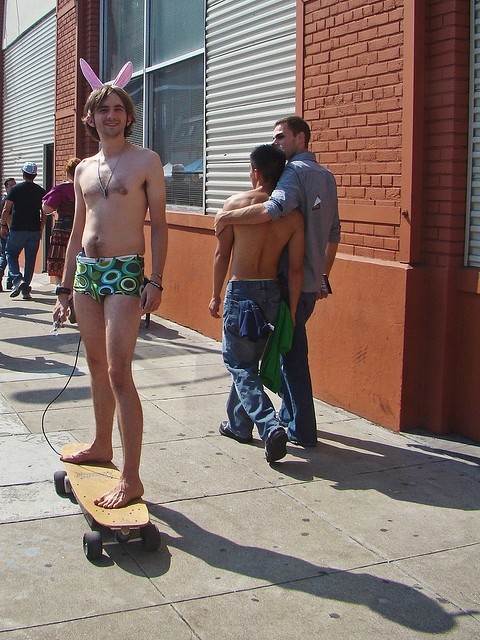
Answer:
[219,420,253,444]
[265,428,288,462]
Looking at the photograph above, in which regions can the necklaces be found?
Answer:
[97,140,126,198]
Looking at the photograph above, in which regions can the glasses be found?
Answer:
[272,133,287,141]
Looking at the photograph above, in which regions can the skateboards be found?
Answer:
[54,442,160,560]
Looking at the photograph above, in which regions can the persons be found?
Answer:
[0,161,47,299]
[214,116,342,448]
[53,86,168,511]
[207,146,304,462]
[43,158,82,323]
[0,177,17,292]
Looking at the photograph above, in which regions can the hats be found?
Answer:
[21,162,37,175]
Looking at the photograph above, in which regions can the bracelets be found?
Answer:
[150,271,163,280]
[149,281,164,290]
[54,287,72,295]
[0,223,5,227]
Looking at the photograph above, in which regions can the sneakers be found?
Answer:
[9,279,27,297]
[22,286,31,299]
